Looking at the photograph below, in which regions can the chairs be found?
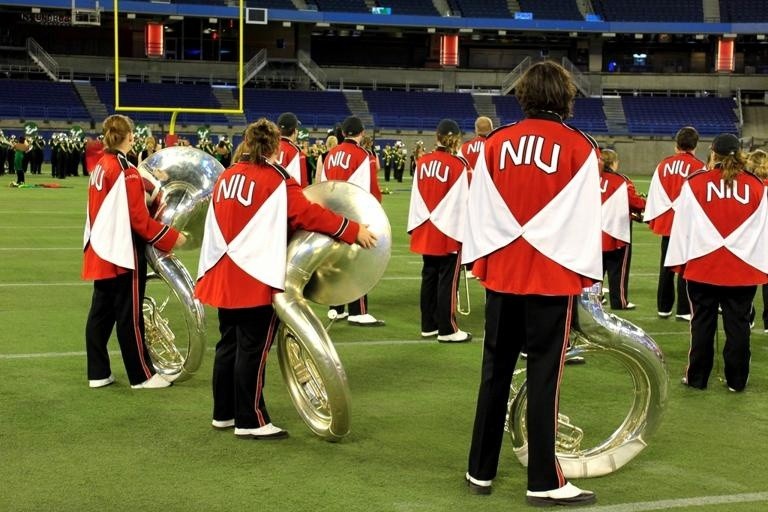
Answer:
[1,1,768,138]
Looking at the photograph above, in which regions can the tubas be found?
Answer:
[137,147,225,382]
[509,287,671,479]
[275,180,391,444]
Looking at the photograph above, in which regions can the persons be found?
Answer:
[409,117,493,341]
[300,137,425,182]
[2,135,104,179]
[14,137,26,187]
[192,121,377,440]
[643,128,706,320]
[127,134,230,168]
[458,63,603,506]
[274,113,312,188]
[80,115,186,388]
[314,117,384,326]
[664,134,768,391]
[600,149,646,309]
[740,149,766,331]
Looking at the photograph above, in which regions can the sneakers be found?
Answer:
[525,480,596,505]
[464,470,493,494]
[681,377,687,384]
[657,310,673,316]
[600,298,608,305]
[436,328,474,343]
[566,356,586,364]
[336,311,379,324]
[749,321,755,328]
[210,417,235,429]
[627,302,636,309]
[419,330,439,336]
[675,314,691,322]
[129,372,173,390]
[88,374,115,389]
[232,422,291,440]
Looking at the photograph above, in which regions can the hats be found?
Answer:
[709,132,741,156]
[340,115,363,136]
[474,116,494,135]
[673,125,700,145]
[436,118,460,136]
[277,112,302,130]
